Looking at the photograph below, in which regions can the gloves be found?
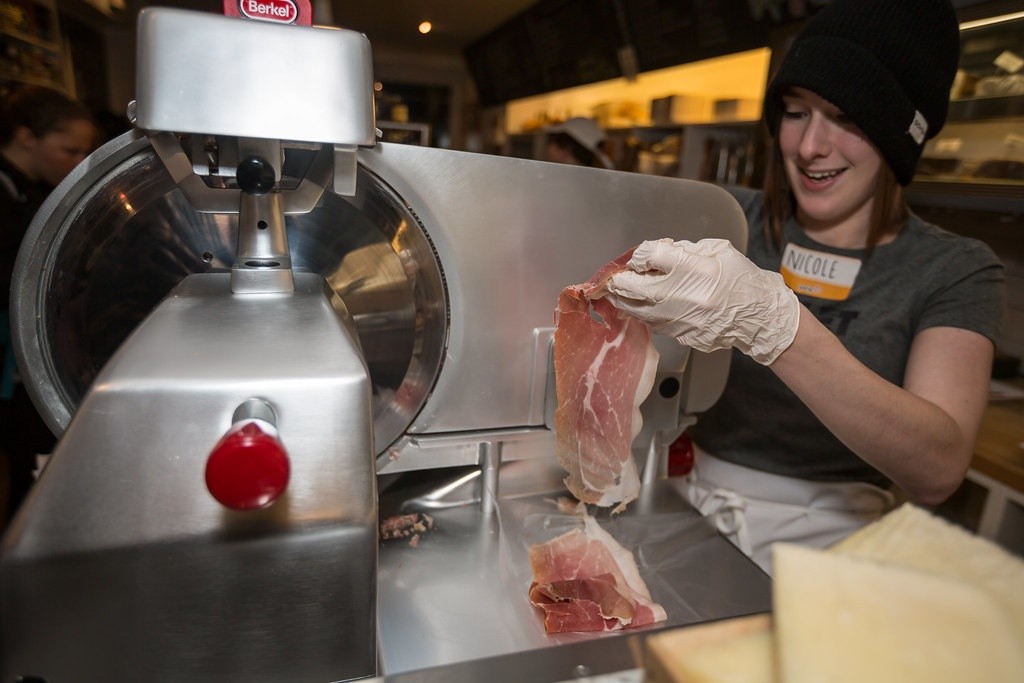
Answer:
[605,238,799,366]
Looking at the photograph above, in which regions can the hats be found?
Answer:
[765,0,960,186]
[545,116,614,170]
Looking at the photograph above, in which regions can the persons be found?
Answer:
[546,130,584,167]
[603,0,1006,586]
[0,80,102,531]
